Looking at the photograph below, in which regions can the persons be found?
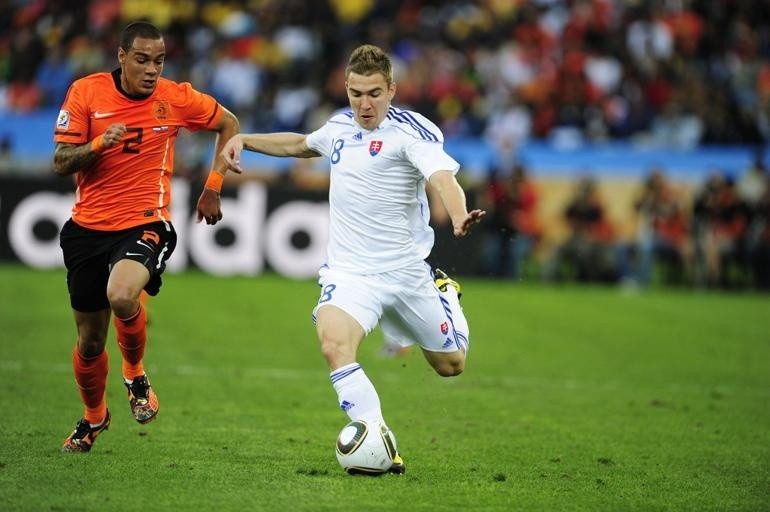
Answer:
[1,1,770,289]
[48,18,241,453]
[221,45,489,477]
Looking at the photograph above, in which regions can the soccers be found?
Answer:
[335,417,396,478]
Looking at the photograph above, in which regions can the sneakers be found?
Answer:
[62,407,112,452]
[122,371,160,424]
[389,450,406,473]
[434,268,462,300]
